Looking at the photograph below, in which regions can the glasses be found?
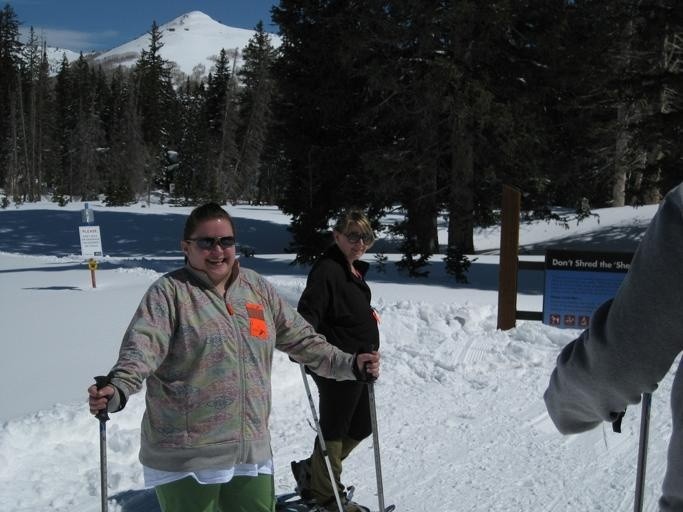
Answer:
[189,231,236,251]
[342,231,374,247]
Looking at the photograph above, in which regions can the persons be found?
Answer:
[544,182,683,512]
[89,203,380,512]
[290,210,380,512]
